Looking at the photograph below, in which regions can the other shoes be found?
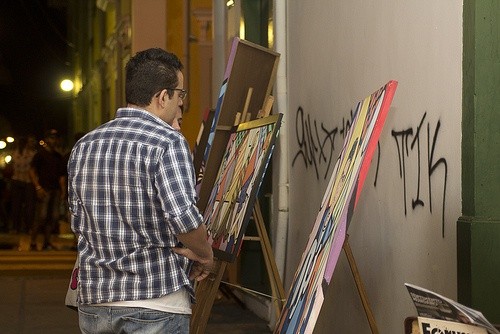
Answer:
[30,244,36,250]
[42,243,54,250]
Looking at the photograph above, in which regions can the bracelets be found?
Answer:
[35,186,42,190]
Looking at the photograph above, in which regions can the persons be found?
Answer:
[0,134,34,250]
[62,131,87,251]
[29,128,60,250]
[64,48,215,334]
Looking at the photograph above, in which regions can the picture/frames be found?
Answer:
[202,113,289,264]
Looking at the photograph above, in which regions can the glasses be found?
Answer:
[156,88,189,100]
